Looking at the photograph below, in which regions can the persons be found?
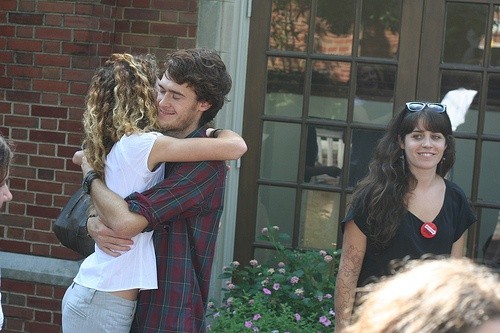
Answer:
[72,48,232,333]
[62,53,248,333]
[262,33,396,252]
[0,138,14,333]
[335,102,477,333]
[342,257,500,333]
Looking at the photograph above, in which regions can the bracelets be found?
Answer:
[213,128,223,138]
[85,215,95,240]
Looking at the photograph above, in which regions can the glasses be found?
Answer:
[405,102,447,113]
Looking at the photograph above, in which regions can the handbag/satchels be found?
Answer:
[53,186,97,258]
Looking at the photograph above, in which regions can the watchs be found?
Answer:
[83,170,99,195]
